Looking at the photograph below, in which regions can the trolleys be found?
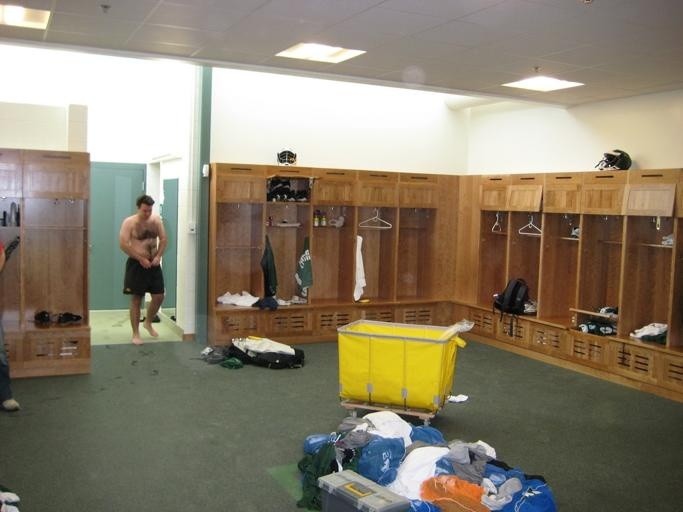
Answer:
[333,315,475,430]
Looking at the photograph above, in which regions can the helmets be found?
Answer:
[275,147,298,167]
[594,148,632,173]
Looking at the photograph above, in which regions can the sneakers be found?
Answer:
[524,299,539,315]
[2,397,22,413]
[31,309,83,327]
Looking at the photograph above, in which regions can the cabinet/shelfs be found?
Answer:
[204,156,457,351]
[458,172,683,408]
[0,146,98,377]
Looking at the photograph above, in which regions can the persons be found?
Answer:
[119,196,167,346]
[1,241,22,412]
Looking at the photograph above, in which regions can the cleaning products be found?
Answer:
[312,209,327,228]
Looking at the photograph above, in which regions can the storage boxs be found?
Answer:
[315,465,412,512]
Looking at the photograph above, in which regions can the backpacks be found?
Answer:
[493,276,529,314]
